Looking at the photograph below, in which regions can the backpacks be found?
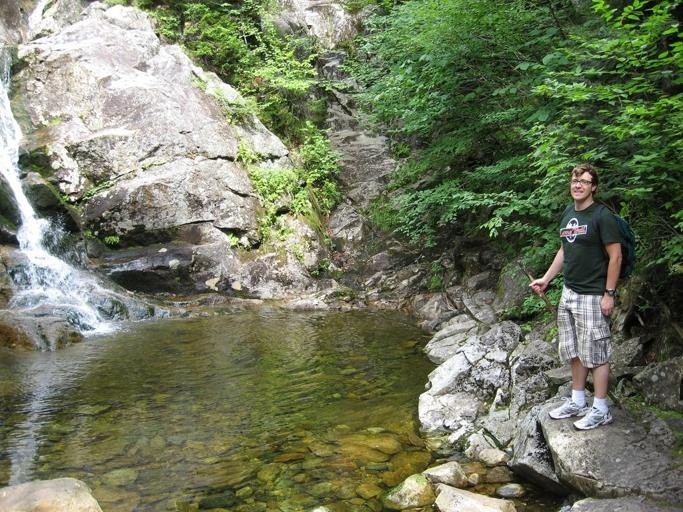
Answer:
[593,205,634,277]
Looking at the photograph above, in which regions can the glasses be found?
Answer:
[572,180,591,184]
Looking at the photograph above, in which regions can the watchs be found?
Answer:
[605,289,616,296]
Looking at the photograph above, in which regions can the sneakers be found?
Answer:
[549,397,588,420]
[573,407,613,430]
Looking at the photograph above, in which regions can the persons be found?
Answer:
[527,166,624,430]
